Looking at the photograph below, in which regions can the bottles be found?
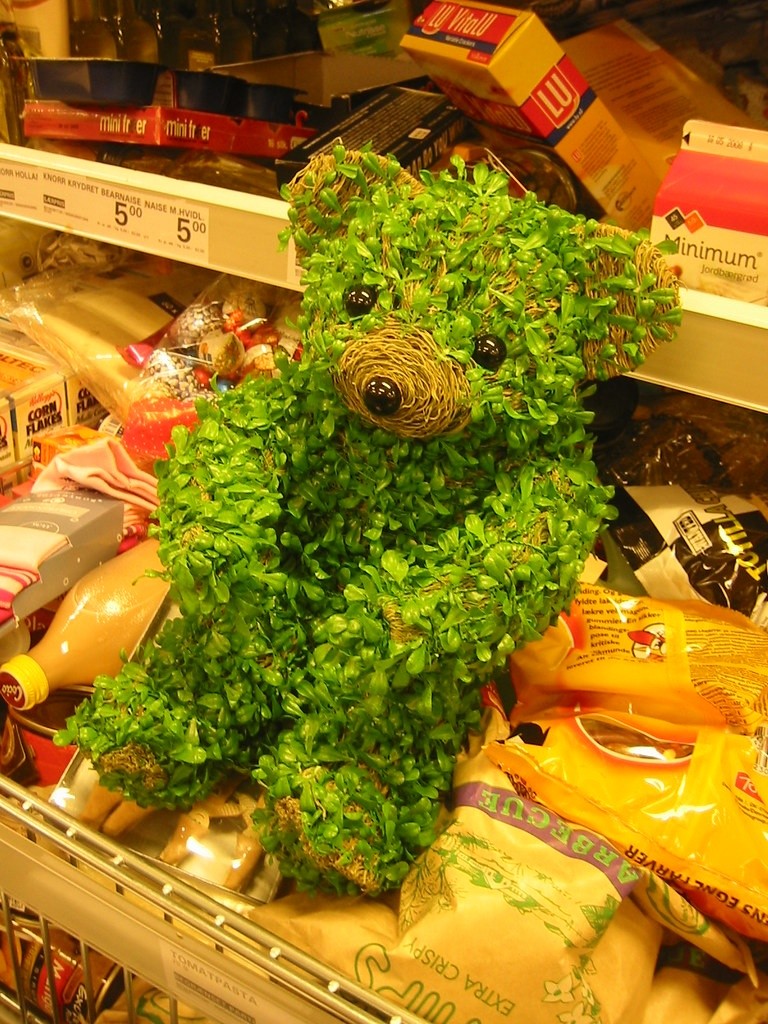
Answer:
[0,537,178,711]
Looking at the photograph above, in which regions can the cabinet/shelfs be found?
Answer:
[0,0,768,1024]
